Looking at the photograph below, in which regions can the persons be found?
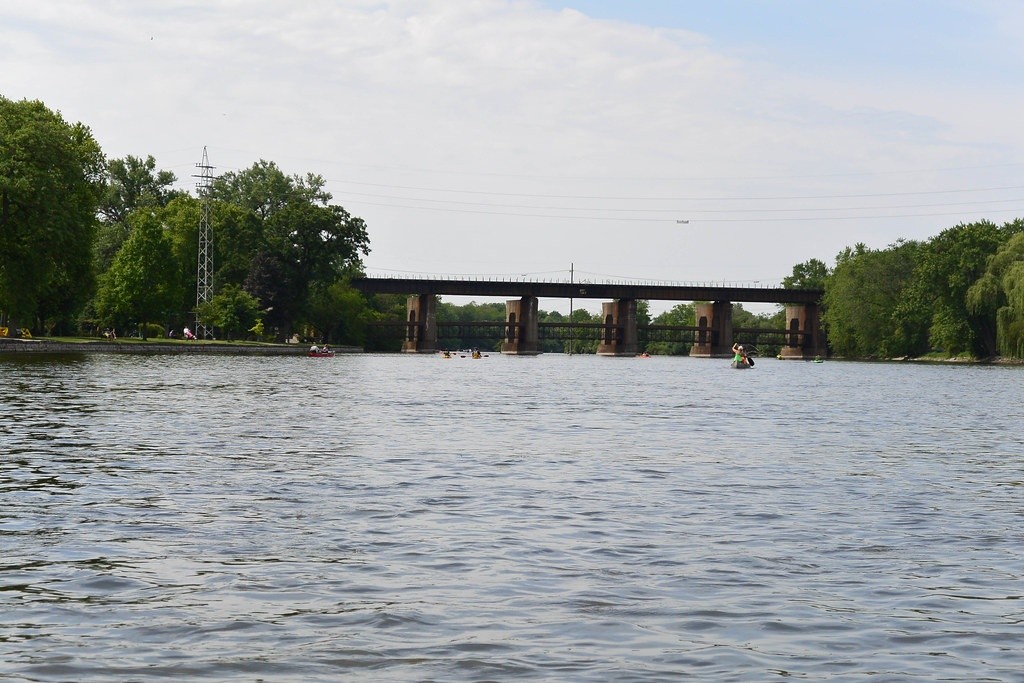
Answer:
[816,353,821,361]
[101,327,116,341]
[732,343,751,365]
[322,344,329,353]
[184,326,197,340]
[643,352,649,357]
[472,347,480,356]
[169,330,174,337]
[310,342,319,353]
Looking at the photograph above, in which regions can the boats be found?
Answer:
[806,359,822,363]
[777,356,784,360]
[731,360,750,368]
[307,351,335,357]
[442,356,451,358]
[637,354,651,358]
[471,353,482,359]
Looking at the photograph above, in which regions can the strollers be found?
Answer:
[185,330,196,340]
